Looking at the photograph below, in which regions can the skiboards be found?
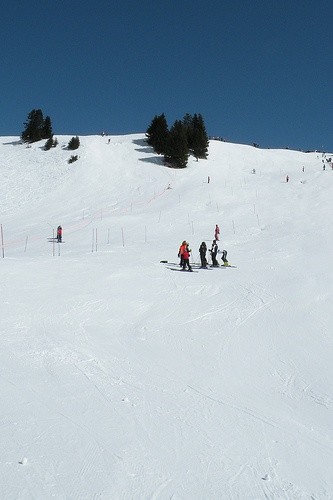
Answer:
[166,262,237,273]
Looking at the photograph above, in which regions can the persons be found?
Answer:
[287,176,289,182]
[57,225,62,242]
[208,176,210,183]
[217,250,228,265]
[209,240,218,265]
[214,225,220,240]
[199,242,208,268]
[178,241,192,271]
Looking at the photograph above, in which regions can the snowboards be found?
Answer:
[48,241,64,243]
[48,237,62,239]
